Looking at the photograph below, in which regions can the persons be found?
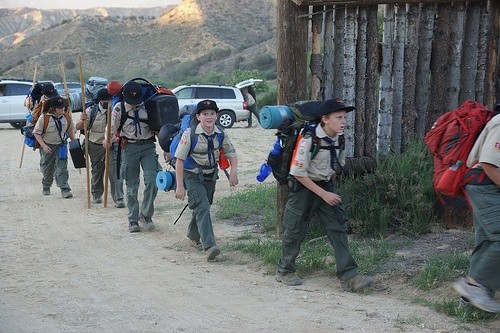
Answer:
[243,89,261,128]
[453,113,499,316]
[175,101,238,260]
[27,80,171,232]
[275,99,372,292]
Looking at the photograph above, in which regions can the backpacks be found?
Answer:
[111,77,168,144]
[84,75,108,99]
[107,80,120,94]
[79,101,101,136]
[169,104,196,170]
[31,98,71,126]
[26,80,55,111]
[267,100,345,192]
[422,99,500,209]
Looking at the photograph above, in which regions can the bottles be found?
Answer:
[271,140,283,154]
[450,160,463,171]
[256,164,272,183]
[59,141,67,160]
[218,150,230,169]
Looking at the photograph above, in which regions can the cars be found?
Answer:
[54,82,87,111]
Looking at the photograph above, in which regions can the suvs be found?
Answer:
[170,78,263,129]
[0,77,43,129]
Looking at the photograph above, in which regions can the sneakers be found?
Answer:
[128,221,139,232]
[451,276,500,314]
[140,212,155,229]
[275,268,302,286]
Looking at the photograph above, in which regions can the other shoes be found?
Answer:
[61,188,72,198]
[42,188,50,195]
[93,195,102,203]
[191,239,202,247]
[205,246,220,260]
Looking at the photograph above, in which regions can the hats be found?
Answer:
[97,89,112,100]
[41,84,58,98]
[318,98,357,116]
[50,97,67,107]
[197,100,219,114]
[123,81,142,104]
